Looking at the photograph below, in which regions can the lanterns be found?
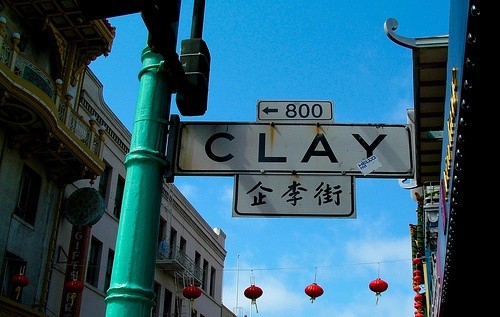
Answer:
[183,270,202,313]
[413,255,425,317]
[245,271,263,313]
[369,264,388,304]
[10,261,30,299]
[304,266,325,303]
[66,262,84,308]
